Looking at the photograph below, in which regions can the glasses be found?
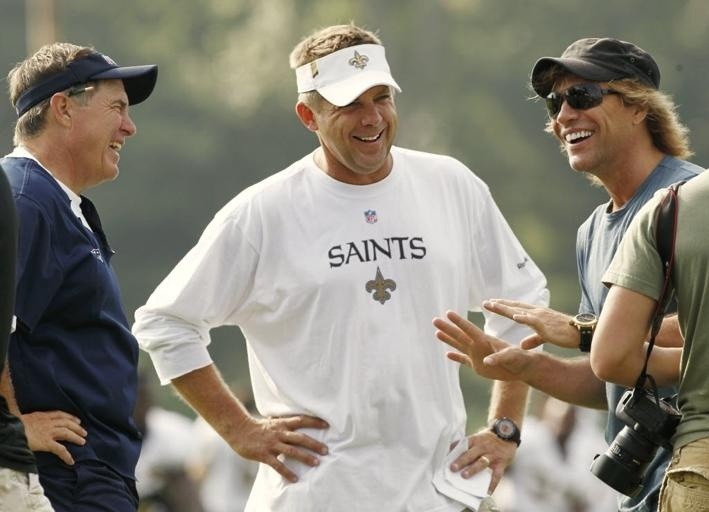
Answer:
[546,86,622,119]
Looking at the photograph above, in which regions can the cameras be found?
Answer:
[588,389,683,499]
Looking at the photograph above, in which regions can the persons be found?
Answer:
[434,38,709,512]
[129,22,554,512]
[0,165,52,512]
[2,42,159,512]
[589,167,709,511]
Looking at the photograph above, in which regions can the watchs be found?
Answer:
[568,313,597,352]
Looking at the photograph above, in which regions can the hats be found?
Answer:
[531,38,661,98]
[13,52,158,116]
[296,43,401,106]
